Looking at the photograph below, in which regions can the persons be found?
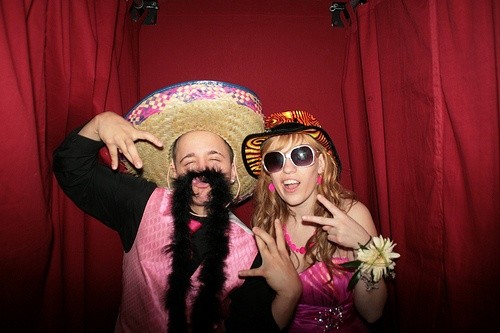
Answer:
[50,80,303,333]
[241,111,387,333]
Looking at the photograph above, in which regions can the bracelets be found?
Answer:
[353,235,372,251]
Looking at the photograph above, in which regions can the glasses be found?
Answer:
[261,144,321,173]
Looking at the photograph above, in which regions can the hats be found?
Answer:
[241,111,343,181]
[117,80,267,206]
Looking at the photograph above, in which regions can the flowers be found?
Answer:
[339,235,401,293]
